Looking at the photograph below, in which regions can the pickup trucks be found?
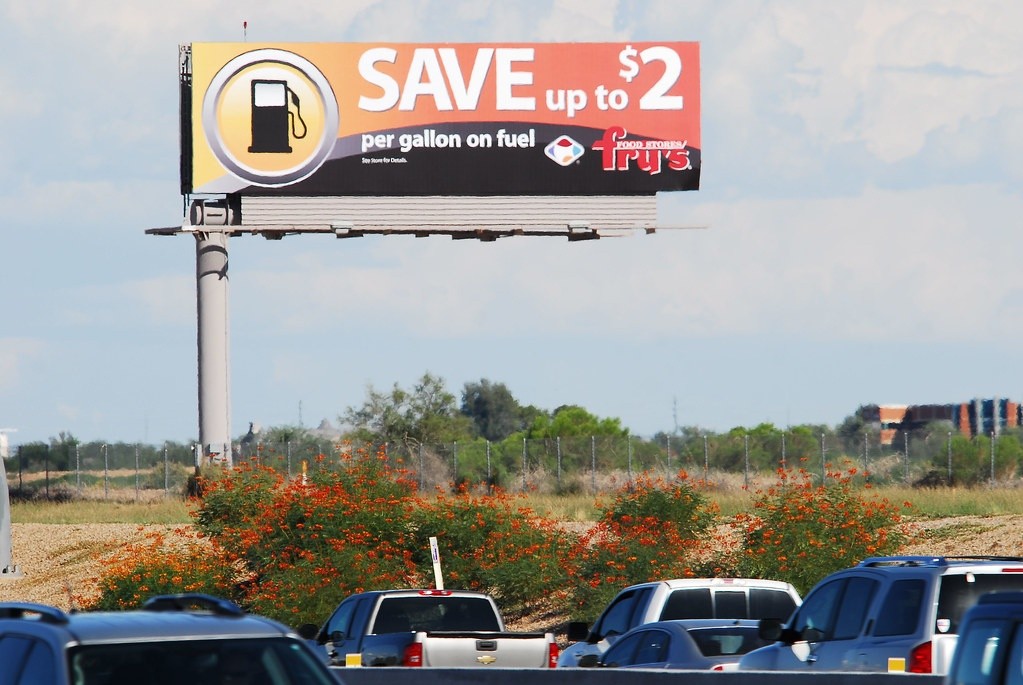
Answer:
[295,587,562,670]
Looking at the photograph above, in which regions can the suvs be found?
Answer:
[944,589,1023,685]
[0,592,348,685]
[556,578,803,669]
[733,552,1023,685]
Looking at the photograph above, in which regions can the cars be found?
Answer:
[592,618,786,677]
